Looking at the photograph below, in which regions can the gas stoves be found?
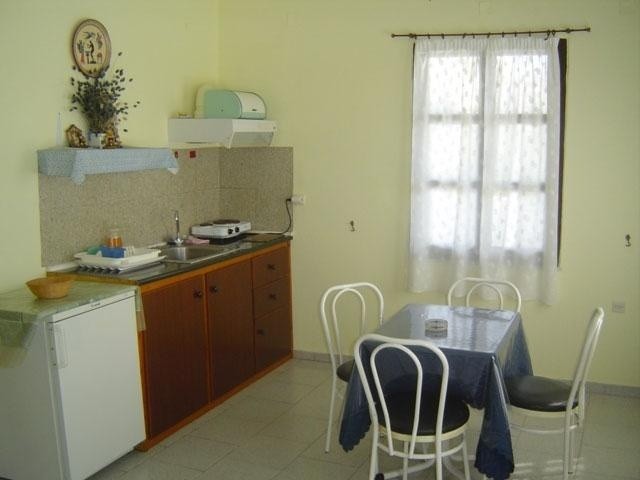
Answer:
[192,218,251,239]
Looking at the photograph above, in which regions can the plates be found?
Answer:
[424,319,449,332]
[84,246,101,255]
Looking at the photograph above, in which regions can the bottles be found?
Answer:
[109,229,123,247]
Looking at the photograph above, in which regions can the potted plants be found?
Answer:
[66,50,143,148]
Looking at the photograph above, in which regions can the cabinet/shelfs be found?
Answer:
[251,245,294,371]
[141,258,253,444]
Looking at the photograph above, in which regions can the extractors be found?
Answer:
[167,118,276,149]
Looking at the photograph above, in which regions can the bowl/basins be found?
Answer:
[101,246,125,257]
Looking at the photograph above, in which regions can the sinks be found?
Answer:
[159,247,240,264]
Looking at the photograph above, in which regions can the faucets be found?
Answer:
[175,210,179,242]
